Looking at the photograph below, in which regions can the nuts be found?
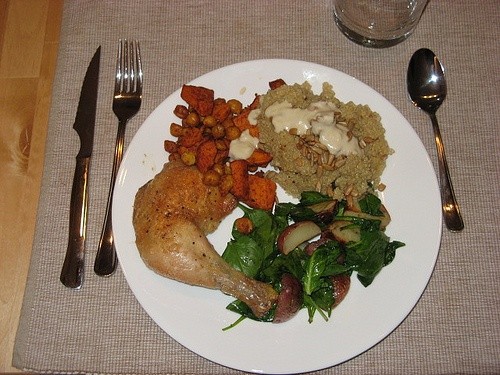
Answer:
[297,111,385,208]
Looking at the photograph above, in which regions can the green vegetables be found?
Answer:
[221,191,405,331]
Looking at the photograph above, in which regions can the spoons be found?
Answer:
[405,48,466,234]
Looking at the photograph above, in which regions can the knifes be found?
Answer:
[59,45,101,290]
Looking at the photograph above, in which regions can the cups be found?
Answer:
[331,0,429,49]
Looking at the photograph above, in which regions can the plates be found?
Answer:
[111,59,443,375]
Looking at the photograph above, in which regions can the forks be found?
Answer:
[94,37,145,278]
